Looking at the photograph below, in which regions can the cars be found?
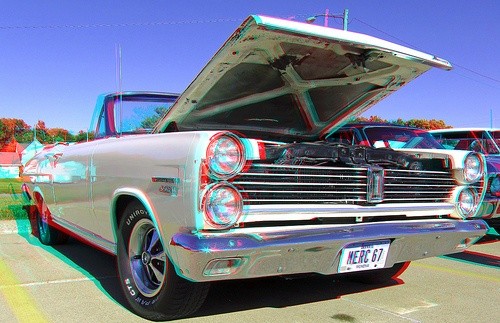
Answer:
[427,128,500,220]
[325,123,446,154]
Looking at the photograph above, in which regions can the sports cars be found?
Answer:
[19,14,488,322]
